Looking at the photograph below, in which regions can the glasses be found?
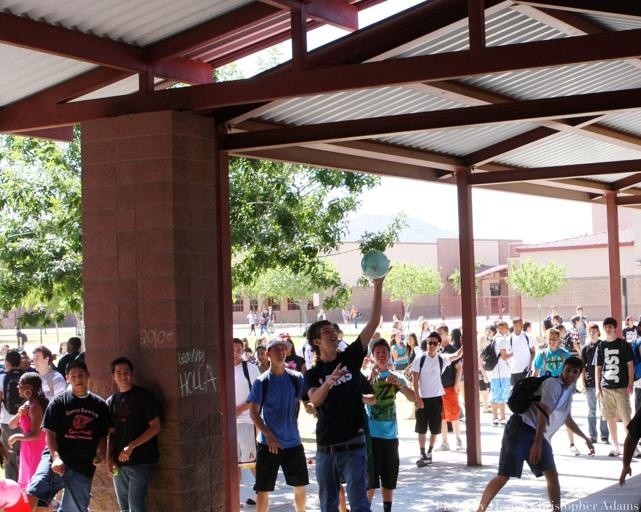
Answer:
[427,341,440,346]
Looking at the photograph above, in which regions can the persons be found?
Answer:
[474,306,641,512]
[0,337,163,512]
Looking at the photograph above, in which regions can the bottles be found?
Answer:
[110,464,119,475]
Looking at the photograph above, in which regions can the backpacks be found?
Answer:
[480,336,502,372]
[507,370,552,427]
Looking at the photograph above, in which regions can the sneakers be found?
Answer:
[415,419,641,467]
[247,493,258,505]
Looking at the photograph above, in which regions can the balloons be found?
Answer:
[360,250,388,281]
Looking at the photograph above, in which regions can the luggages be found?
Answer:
[2,368,28,415]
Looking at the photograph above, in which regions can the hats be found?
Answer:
[265,338,292,351]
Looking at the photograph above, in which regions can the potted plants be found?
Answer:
[351,215,411,281]
